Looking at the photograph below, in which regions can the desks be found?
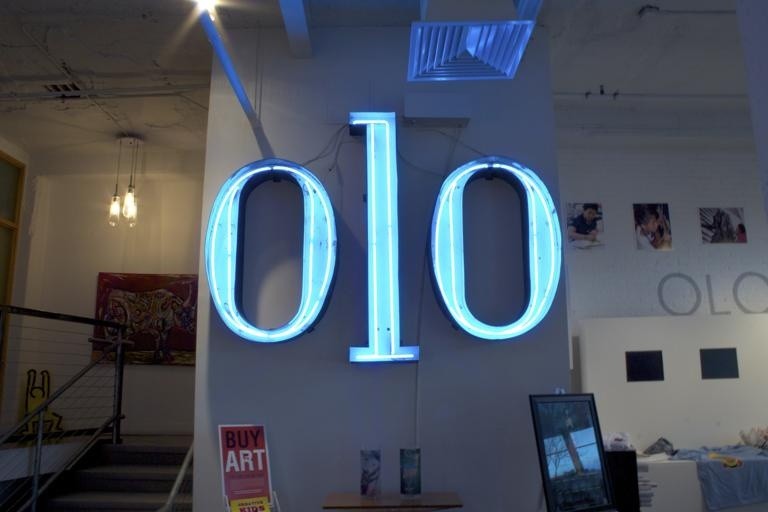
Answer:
[323,492,463,512]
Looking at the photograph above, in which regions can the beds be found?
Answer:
[636,446,768,512]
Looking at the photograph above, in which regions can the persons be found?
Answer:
[733,222,746,243]
[710,207,732,242]
[634,203,669,251]
[565,203,602,243]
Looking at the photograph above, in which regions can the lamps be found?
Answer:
[107,135,143,227]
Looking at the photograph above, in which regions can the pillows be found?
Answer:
[604,432,633,450]
